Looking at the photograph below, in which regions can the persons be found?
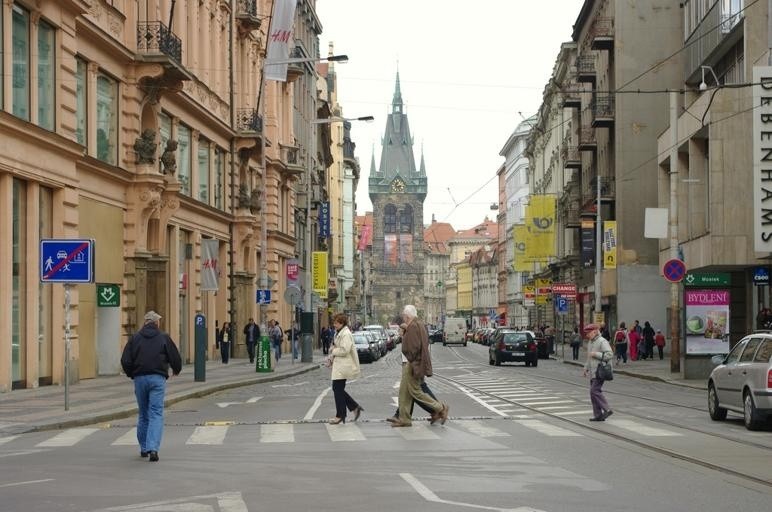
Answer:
[218,322,233,364]
[267,319,283,363]
[544,326,552,335]
[325,314,364,425]
[582,324,614,422]
[390,305,449,427]
[756,308,772,330]
[285,326,301,359]
[321,326,334,354]
[244,318,260,364]
[600,319,666,364]
[120,310,182,461]
[571,328,581,359]
[386,323,441,424]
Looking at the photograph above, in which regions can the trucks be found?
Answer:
[441,317,468,347]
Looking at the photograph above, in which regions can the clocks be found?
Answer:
[392,177,407,193]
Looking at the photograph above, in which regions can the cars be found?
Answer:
[428,328,444,345]
[363,322,402,357]
[706,329,772,432]
[487,331,539,369]
[350,330,374,364]
[516,329,552,359]
[466,326,518,346]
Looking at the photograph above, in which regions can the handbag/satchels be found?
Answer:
[595,351,613,382]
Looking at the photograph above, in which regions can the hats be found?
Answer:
[583,323,601,331]
[143,310,163,326]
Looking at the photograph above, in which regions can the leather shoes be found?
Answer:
[438,403,449,425]
[391,420,412,427]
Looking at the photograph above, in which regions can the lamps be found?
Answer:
[698,64,720,92]
[310,114,377,128]
[263,52,352,67]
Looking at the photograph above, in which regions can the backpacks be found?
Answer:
[615,328,627,343]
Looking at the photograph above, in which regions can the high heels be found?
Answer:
[329,417,346,425]
[349,405,364,423]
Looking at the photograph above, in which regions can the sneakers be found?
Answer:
[430,412,440,425]
[589,413,605,421]
[141,451,148,457]
[149,451,159,461]
[599,409,614,421]
[386,415,401,423]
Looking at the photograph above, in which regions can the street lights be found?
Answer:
[299,114,375,364]
[255,53,350,373]
